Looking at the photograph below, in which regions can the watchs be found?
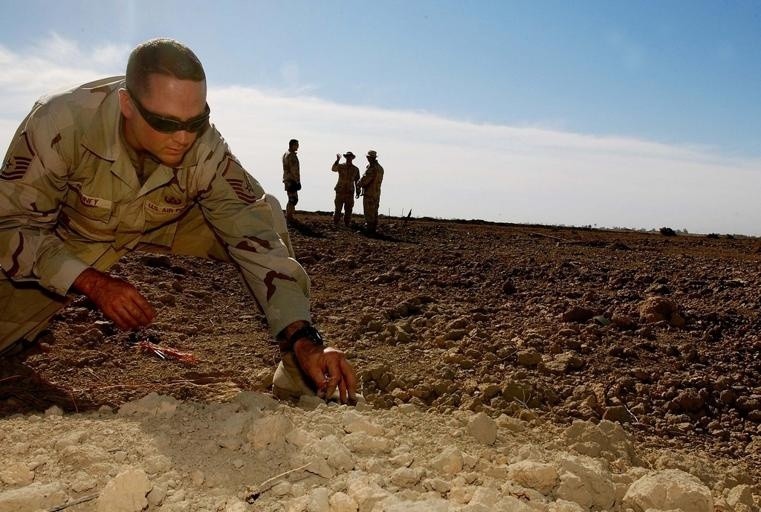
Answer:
[287,326,324,349]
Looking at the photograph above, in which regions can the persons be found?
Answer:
[282,139,302,224]
[356,150,384,237]
[0,38,358,408]
[332,152,361,229]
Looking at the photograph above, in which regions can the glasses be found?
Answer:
[128,86,211,134]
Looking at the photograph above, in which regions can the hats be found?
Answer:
[343,152,355,159]
[366,151,377,158]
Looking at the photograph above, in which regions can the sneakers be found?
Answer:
[272,360,366,406]
